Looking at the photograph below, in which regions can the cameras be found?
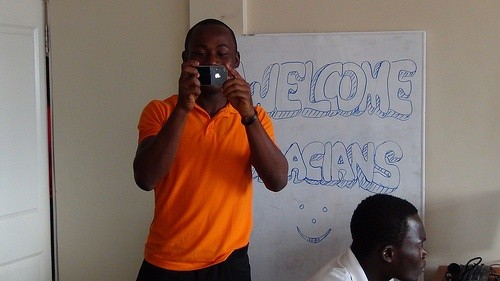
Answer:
[189,65,228,87]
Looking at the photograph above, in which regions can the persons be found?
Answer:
[129,18,289,281]
[299,190,433,281]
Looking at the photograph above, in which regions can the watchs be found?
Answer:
[241,110,258,127]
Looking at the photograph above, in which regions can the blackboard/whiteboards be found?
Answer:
[233,32,427,280]
